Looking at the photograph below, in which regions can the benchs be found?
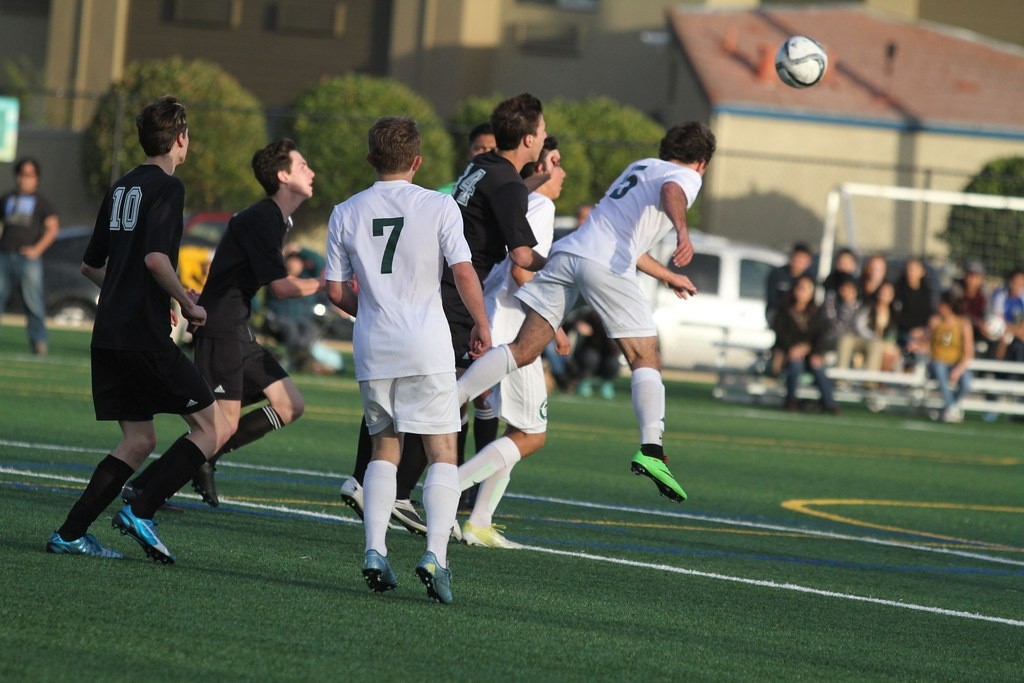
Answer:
[692,341,1022,416]
[0,313,719,384]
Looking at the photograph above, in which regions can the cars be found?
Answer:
[551,215,799,374]
[2,208,358,342]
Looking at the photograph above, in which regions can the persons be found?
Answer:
[47,96,233,564]
[575,202,594,226]
[454,119,716,502]
[765,244,1024,425]
[249,244,358,377]
[0,157,66,355]
[165,136,354,508]
[542,292,625,401]
[322,91,564,602]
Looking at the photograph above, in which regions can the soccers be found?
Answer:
[774,34,830,89]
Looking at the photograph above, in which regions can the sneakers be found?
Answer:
[448,520,463,542]
[110,504,174,566]
[338,477,394,529]
[119,483,182,511]
[362,549,398,592]
[195,461,219,507]
[630,452,686,501]
[390,498,428,537]
[463,520,524,550]
[415,552,452,605]
[46,532,123,560]
